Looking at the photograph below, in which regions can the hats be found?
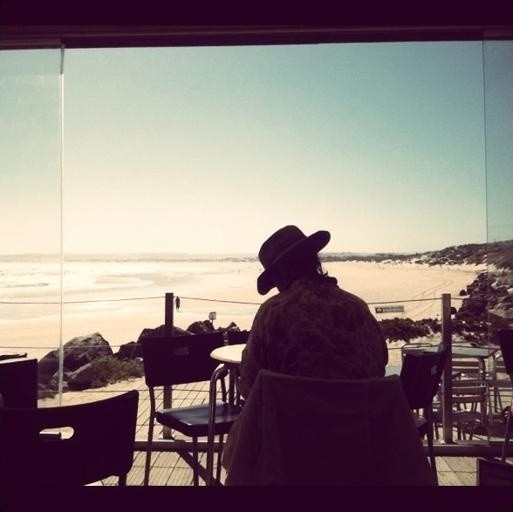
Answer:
[257,225,330,295]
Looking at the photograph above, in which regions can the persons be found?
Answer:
[234,223,390,406]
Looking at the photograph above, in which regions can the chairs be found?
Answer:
[0,330,513,486]
